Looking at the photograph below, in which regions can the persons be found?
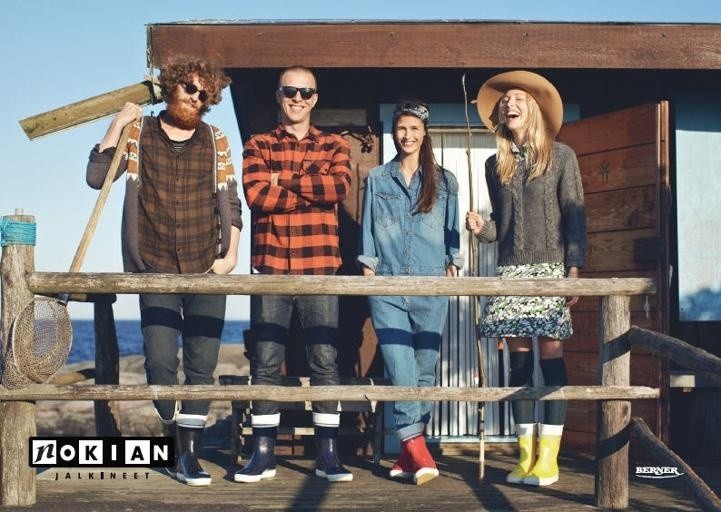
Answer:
[85,54,245,488]
[357,102,466,485]
[234,66,354,483]
[465,70,590,487]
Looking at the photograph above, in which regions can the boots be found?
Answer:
[506,419,537,486]
[389,432,415,484]
[152,398,180,427]
[405,434,440,487]
[171,407,213,487]
[310,409,355,482]
[233,409,281,484]
[522,422,567,486]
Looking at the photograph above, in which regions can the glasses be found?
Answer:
[178,79,208,103]
[280,83,319,99]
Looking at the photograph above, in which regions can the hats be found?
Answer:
[475,70,565,144]
[394,104,430,123]
